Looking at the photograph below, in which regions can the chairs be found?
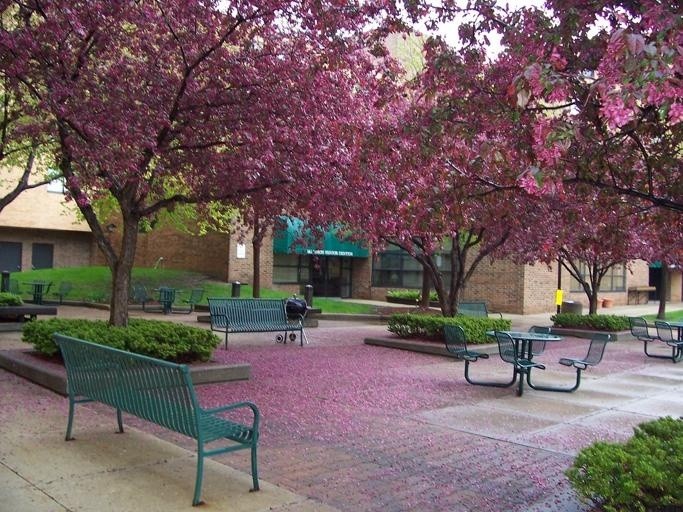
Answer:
[559,332,611,393]
[23,280,53,306]
[505,325,552,383]
[157,288,175,313]
[6,277,23,298]
[131,282,155,310]
[51,279,74,306]
[182,288,205,315]
[655,320,683,363]
[628,316,659,358]
[676,318,683,357]
[494,330,545,396]
[441,324,490,386]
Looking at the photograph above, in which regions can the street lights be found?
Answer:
[554,254,567,315]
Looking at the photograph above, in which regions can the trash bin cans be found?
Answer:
[561,300,583,316]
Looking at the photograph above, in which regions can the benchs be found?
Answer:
[458,301,503,320]
[51,331,260,505]
[206,296,310,351]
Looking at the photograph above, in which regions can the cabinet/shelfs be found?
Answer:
[627,286,656,305]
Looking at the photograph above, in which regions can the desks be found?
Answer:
[153,287,182,314]
[32,283,52,306]
[635,321,683,358]
[485,330,562,393]
